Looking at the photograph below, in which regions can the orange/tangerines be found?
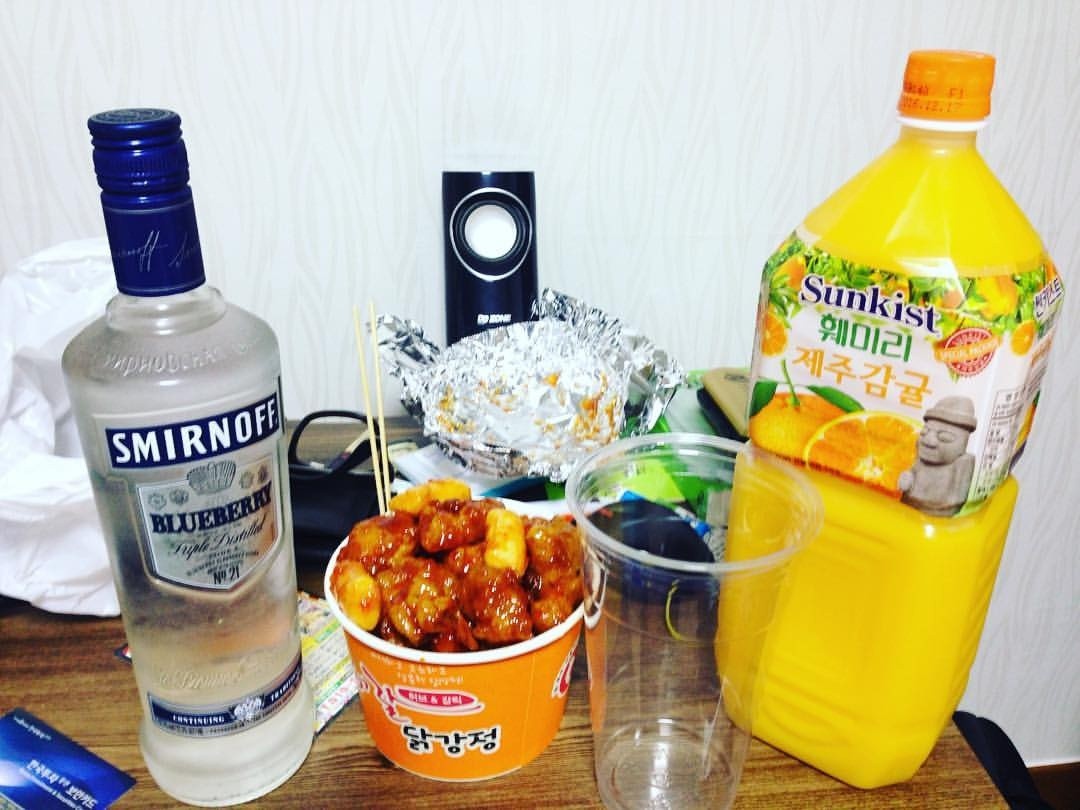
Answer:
[761,240,1036,355]
[749,358,921,493]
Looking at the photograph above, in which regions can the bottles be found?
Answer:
[63,107,316,807]
[712,50,1065,790]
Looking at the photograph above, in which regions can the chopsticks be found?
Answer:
[352,304,392,517]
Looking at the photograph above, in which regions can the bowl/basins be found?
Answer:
[323,496,600,787]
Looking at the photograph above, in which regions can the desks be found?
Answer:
[0,416,1012,810]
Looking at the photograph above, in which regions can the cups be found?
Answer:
[563,432,822,810]
